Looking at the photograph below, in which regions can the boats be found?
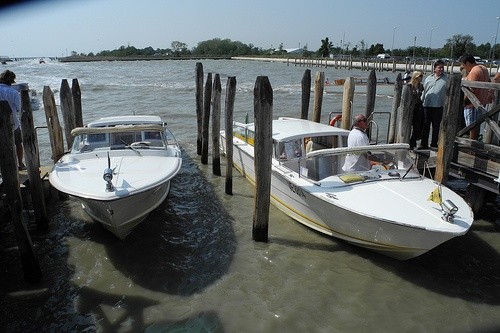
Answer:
[48,115,182,238]
[39,59,45,64]
[219,117,475,261]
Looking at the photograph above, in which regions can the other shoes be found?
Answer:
[18,162,27,171]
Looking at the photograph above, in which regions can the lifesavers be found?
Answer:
[330,114,342,127]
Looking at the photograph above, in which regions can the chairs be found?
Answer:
[306,157,332,181]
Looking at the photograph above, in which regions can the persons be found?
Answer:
[407,71,425,147]
[341,114,372,171]
[421,60,452,148]
[460,55,491,142]
[491,67,500,145]
[0,70,28,171]
